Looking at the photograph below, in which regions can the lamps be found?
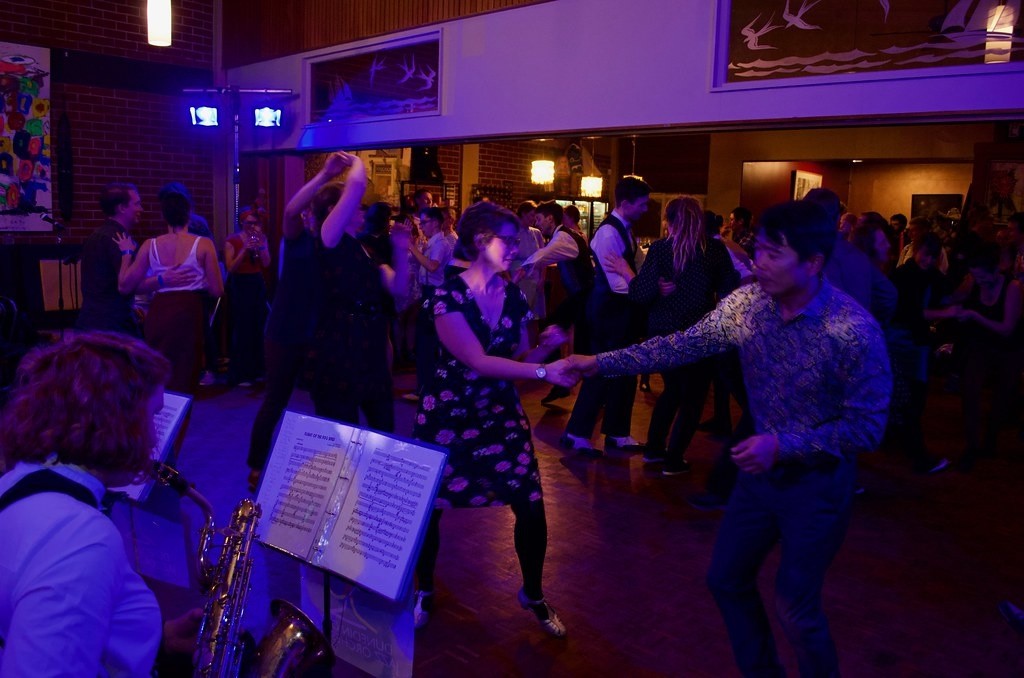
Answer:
[529,135,644,199]
[189,89,284,129]
[144,0,174,48]
[983,0,1018,65]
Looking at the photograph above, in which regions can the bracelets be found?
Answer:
[120,250,136,255]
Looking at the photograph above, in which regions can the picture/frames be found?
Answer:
[793,168,824,202]
[969,142,1024,240]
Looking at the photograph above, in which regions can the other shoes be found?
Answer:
[402,390,420,401]
[686,490,728,511]
[662,457,692,475]
[559,431,608,459]
[605,435,644,451]
[248,369,271,396]
[913,453,952,475]
[541,385,571,403]
[643,442,669,463]
[696,415,730,435]
[640,378,651,392]
[195,371,226,389]
[248,468,260,488]
[957,445,982,475]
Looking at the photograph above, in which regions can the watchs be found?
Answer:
[535,364,546,380]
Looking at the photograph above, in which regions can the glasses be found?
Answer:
[245,222,257,226]
[479,232,522,247]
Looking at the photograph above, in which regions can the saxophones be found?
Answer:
[151,462,336,678]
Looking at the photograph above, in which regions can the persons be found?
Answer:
[697,210,732,442]
[0,331,174,678]
[244,151,460,491]
[563,205,589,249]
[112,183,223,389]
[518,201,545,322]
[728,207,755,259]
[555,177,650,461]
[602,197,740,478]
[226,206,271,377]
[560,201,893,678]
[411,203,579,638]
[85,181,143,332]
[511,203,595,404]
[720,188,1024,484]
[160,183,213,243]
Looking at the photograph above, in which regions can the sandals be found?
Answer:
[413,590,434,629]
[517,588,566,637]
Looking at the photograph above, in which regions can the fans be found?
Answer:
[869,0,1016,53]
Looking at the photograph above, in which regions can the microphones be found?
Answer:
[40,213,64,229]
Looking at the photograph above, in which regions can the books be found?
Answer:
[254,408,451,606]
[106,392,194,507]
